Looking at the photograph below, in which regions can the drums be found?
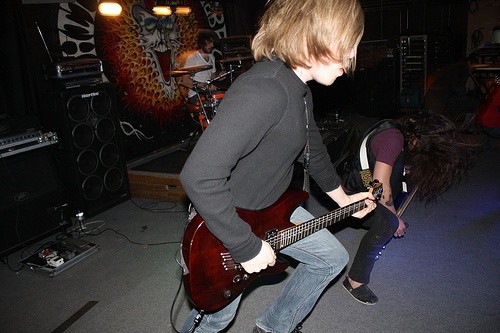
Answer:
[186,91,226,129]
[471,68,500,128]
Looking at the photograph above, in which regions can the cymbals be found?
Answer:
[171,64,214,76]
[224,47,251,52]
[219,56,253,63]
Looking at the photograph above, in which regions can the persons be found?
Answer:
[155,0,377,333]
[311,109,469,306]
[182,32,225,98]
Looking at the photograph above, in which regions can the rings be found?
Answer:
[399,235,401,236]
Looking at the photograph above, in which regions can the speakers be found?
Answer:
[52,82,132,220]
[366,62,399,117]
[0,149,73,259]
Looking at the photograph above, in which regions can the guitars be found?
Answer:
[182,179,384,313]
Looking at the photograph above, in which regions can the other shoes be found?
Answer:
[342,276,378,305]
[253,326,302,333]
